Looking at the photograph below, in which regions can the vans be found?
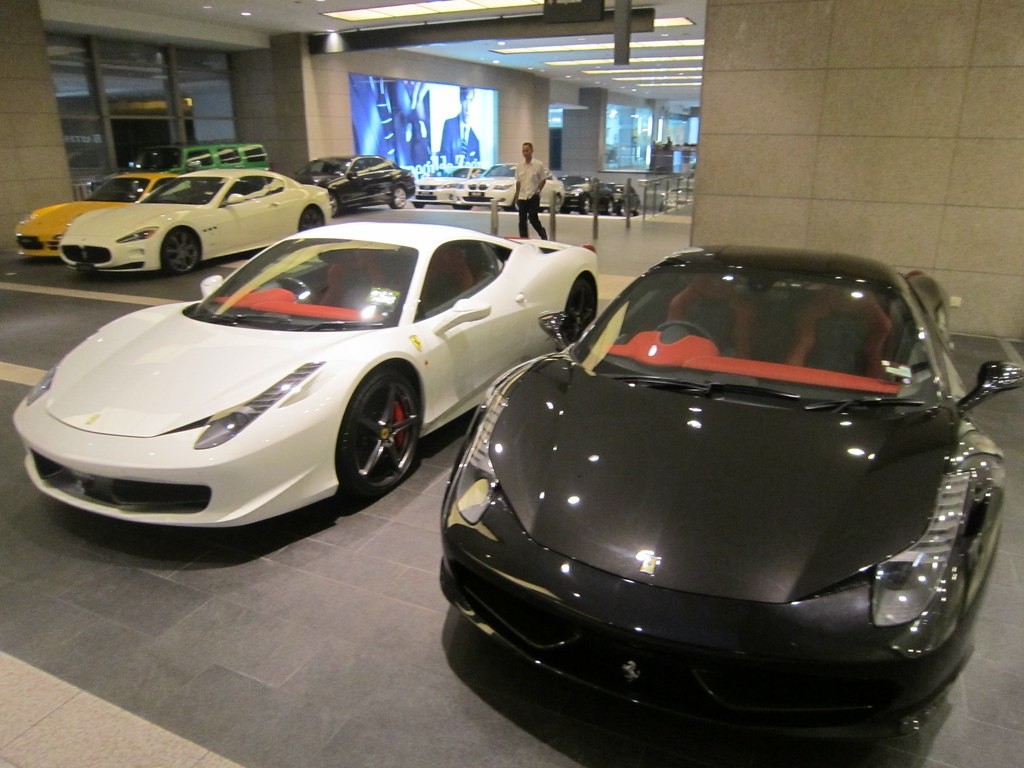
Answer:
[135,139,273,172]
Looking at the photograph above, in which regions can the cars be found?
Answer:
[408,166,487,210]
[455,163,565,213]
[56,170,332,276]
[290,156,416,218]
[659,188,693,213]
[607,183,641,216]
[556,175,614,215]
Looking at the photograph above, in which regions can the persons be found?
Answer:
[438,85,480,165]
[513,142,554,241]
[664,136,673,145]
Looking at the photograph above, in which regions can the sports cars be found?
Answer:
[438,242,1024,734]
[12,221,599,528]
[12,172,182,259]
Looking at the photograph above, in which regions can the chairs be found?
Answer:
[665,270,752,360]
[783,285,894,381]
[390,246,472,309]
[319,249,384,314]
